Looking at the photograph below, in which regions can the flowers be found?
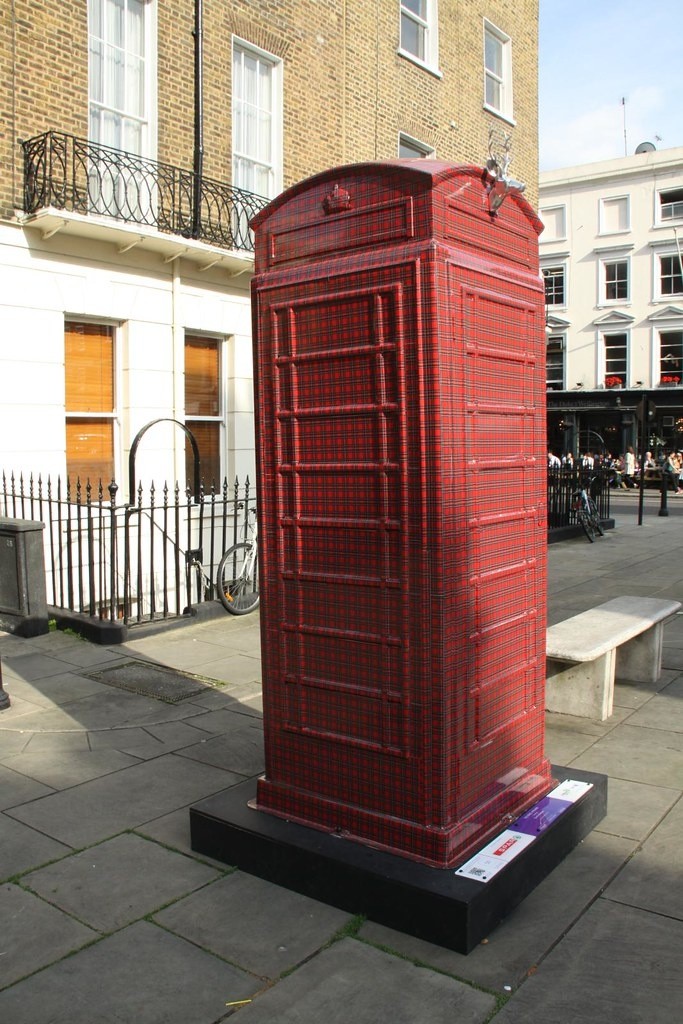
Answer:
[661,376,680,387]
[605,376,623,389]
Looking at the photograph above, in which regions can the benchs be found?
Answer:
[546,596,682,722]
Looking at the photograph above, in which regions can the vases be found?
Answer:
[606,384,622,389]
[664,382,677,387]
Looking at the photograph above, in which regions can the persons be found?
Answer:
[547,446,683,495]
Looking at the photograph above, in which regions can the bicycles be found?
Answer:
[215,505,259,617]
[570,484,605,543]
